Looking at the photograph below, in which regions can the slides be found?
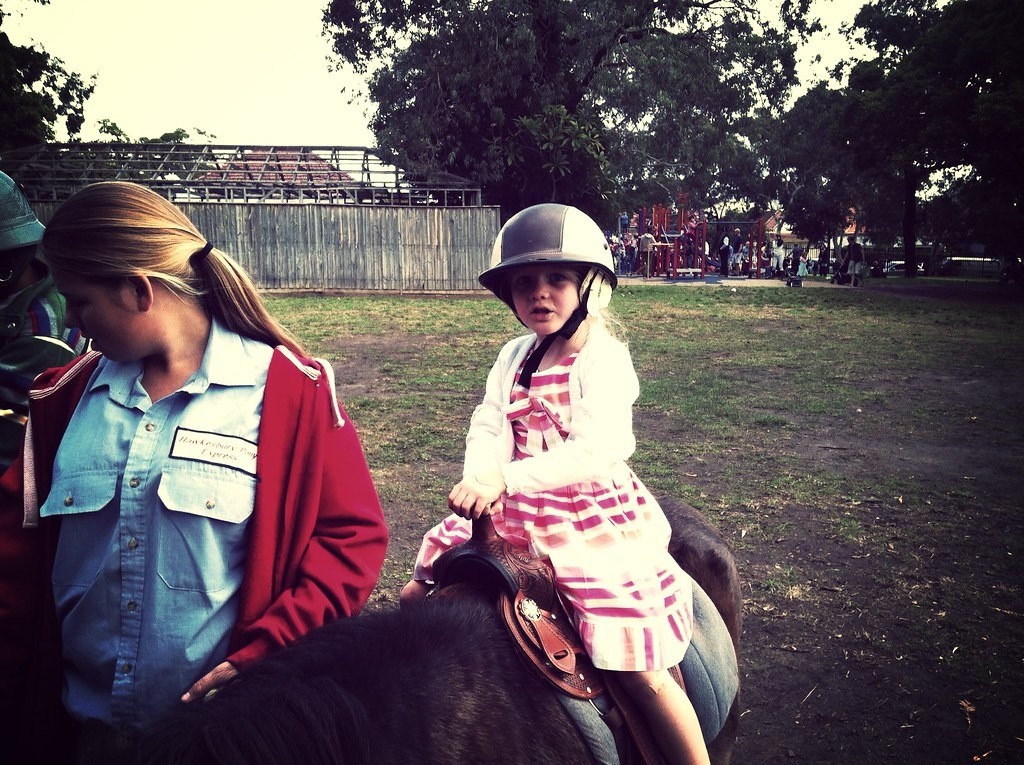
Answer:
[691,244,724,270]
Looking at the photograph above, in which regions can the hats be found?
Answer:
[0,171,46,249]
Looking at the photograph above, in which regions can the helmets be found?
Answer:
[477,203,618,306]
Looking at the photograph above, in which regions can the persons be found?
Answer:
[401,204,711,764]
[0,169,388,747]
[841,236,866,287]
[610,212,828,286]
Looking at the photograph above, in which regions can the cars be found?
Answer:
[882,263,926,278]
